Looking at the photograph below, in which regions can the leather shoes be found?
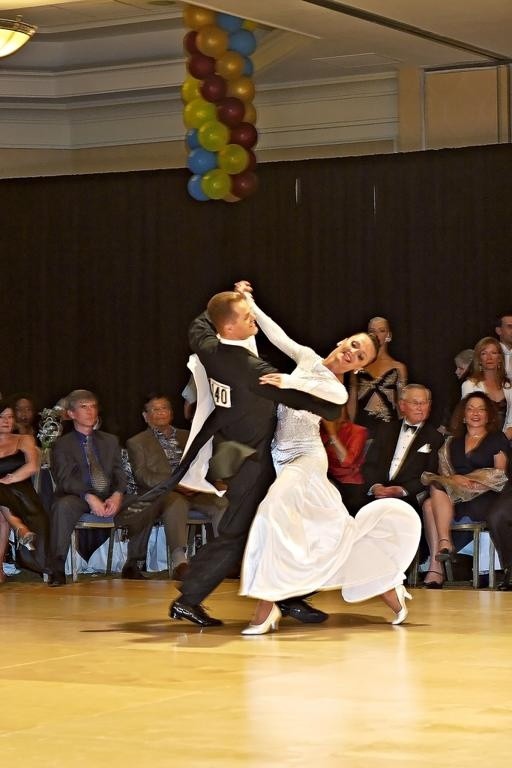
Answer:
[496,567,512,590]
[170,600,223,627]
[48,571,66,587]
[276,600,328,623]
[173,563,189,581]
[122,564,149,579]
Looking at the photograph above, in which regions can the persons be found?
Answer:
[320,310,511,591]
[1,390,229,587]
[113,291,342,628]
[178,279,422,633]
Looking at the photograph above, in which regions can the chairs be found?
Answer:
[406,519,496,590]
[32,447,211,583]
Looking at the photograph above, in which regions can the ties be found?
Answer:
[86,435,106,492]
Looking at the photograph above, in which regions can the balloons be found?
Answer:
[178,5,260,202]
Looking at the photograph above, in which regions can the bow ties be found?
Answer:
[404,424,418,433]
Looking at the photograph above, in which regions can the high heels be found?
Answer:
[391,585,412,625]
[240,602,282,635]
[435,539,457,564]
[422,571,445,589]
[16,527,35,552]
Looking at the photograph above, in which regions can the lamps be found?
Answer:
[0,15,38,57]
